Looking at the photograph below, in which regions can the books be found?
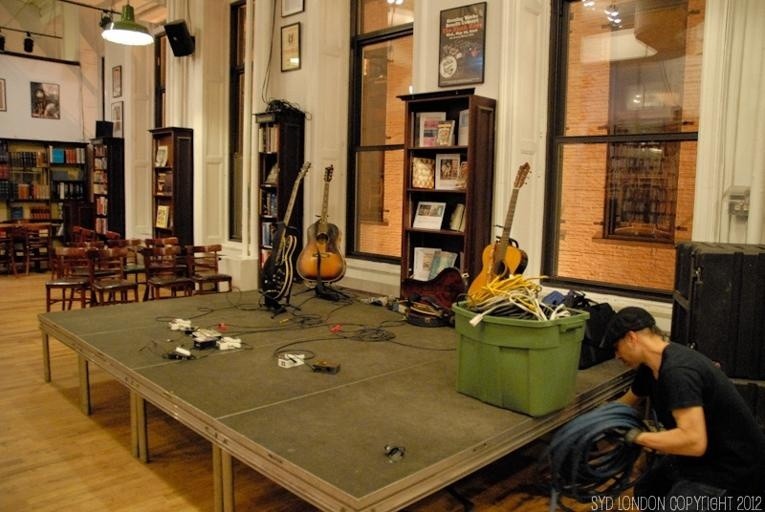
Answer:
[262,223,279,249]
[413,199,465,232]
[413,247,458,281]
[415,107,469,146]
[257,124,279,153]
[258,187,278,216]
[0,145,108,235]
[412,153,468,191]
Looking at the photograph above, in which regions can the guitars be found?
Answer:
[466,162,533,298]
[296,164,347,284]
[257,163,311,300]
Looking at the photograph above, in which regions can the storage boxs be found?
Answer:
[451,300,591,418]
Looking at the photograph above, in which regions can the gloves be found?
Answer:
[601,426,642,444]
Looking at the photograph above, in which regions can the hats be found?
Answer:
[598,306,656,362]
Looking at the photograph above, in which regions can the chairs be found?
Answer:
[0,229,232,312]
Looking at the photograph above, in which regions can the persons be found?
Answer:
[599,306,765,512]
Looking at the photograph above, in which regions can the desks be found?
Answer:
[38,285,639,508]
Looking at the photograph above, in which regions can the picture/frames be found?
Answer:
[280,0,304,73]
[111,65,124,139]
[0,78,7,112]
[30,81,60,119]
[437,2,487,88]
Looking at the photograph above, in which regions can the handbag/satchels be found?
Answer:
[573,301,617,368]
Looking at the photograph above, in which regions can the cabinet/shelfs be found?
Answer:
[252,111,306,289]
[147,127,193,291]
[0,137,126,273]
[400,96,497,311]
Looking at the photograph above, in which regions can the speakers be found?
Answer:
[164,18,195,57]
[96,120,113,137]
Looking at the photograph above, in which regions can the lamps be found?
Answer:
[100,0,153,47]
[0,26,63,53]
[59,0,123,30]
